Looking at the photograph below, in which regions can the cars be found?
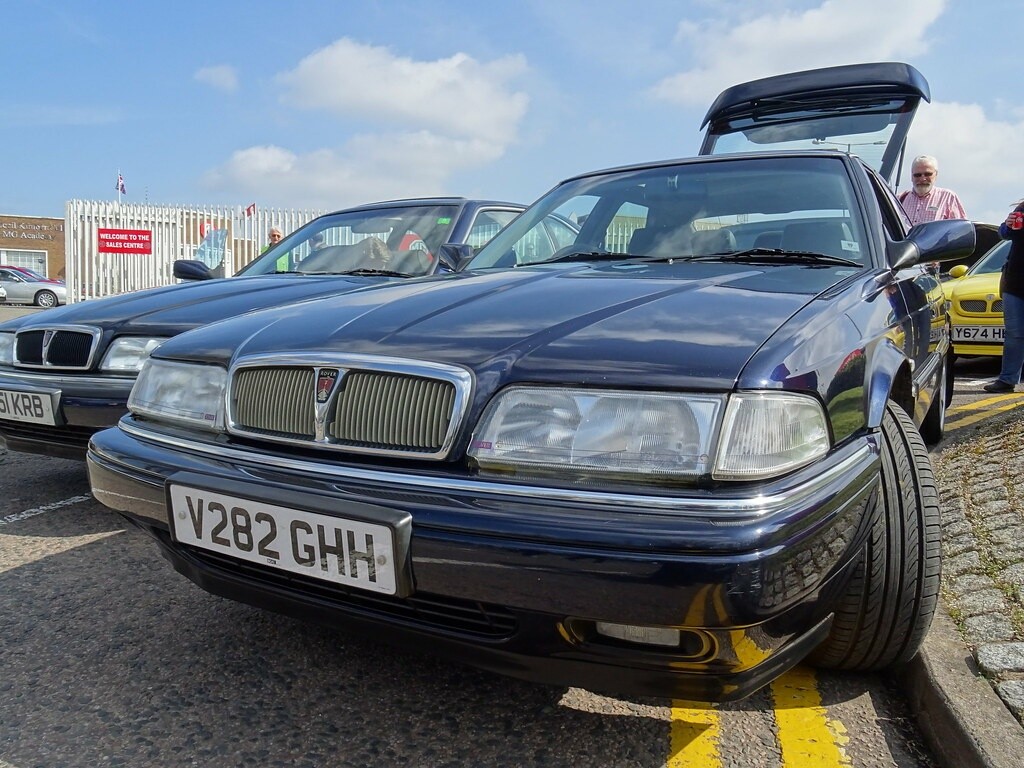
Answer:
[949,238,1016,356]
[86,62,944,704]
[0,266,66,307]
[0,196,600,471]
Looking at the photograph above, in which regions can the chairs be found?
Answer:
[777,221,859,259]
[698,229,737,254]
[627,225,698,258]
[296,243,430,274]
[752,230,783,249]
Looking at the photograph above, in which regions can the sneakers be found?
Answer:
[984,380,1015,392]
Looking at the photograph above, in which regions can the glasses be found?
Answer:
[913,171,935,177]
[272,234,280,238]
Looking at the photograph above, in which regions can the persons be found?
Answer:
[895,153,967,276]
[885,280,932,369]
[262,226,330,270]
[983,198,1024,394]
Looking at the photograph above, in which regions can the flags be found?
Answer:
[115,174,127,194]
[241,203,256,220]
